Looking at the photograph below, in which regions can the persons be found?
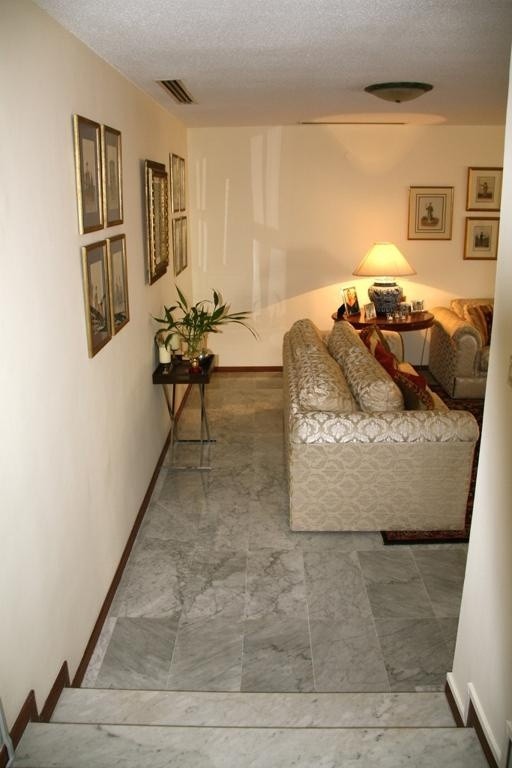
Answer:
[426,201,436,223]
[345,290,357,312]
[479,230,484,245]
[480,181,488,197]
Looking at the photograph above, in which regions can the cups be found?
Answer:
[385,300,424,320]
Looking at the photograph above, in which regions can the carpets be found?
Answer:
[377,361,487,546]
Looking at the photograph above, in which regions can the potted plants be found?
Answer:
[152,283,259,363]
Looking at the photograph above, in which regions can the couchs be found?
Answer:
[276,314,481,535]
[430,294,496,403]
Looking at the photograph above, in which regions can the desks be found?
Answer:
[330,305,434,369]
[151,353,221,472]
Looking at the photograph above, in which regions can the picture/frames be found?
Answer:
[340,285,363,318]
[405,182,456,242]
[70,109,133,361]
[167,149,191,278]
[142,156,172,286]
[465,165,504,213]
[462,215,500,261]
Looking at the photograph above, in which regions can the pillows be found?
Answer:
[359,323,390,353]
[393,370,427,389]
[463,301,493,347]
[383,362,434,409]
[373,339,396,370]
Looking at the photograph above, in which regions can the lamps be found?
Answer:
[351,238,418,318]
[361,80,434,106]
